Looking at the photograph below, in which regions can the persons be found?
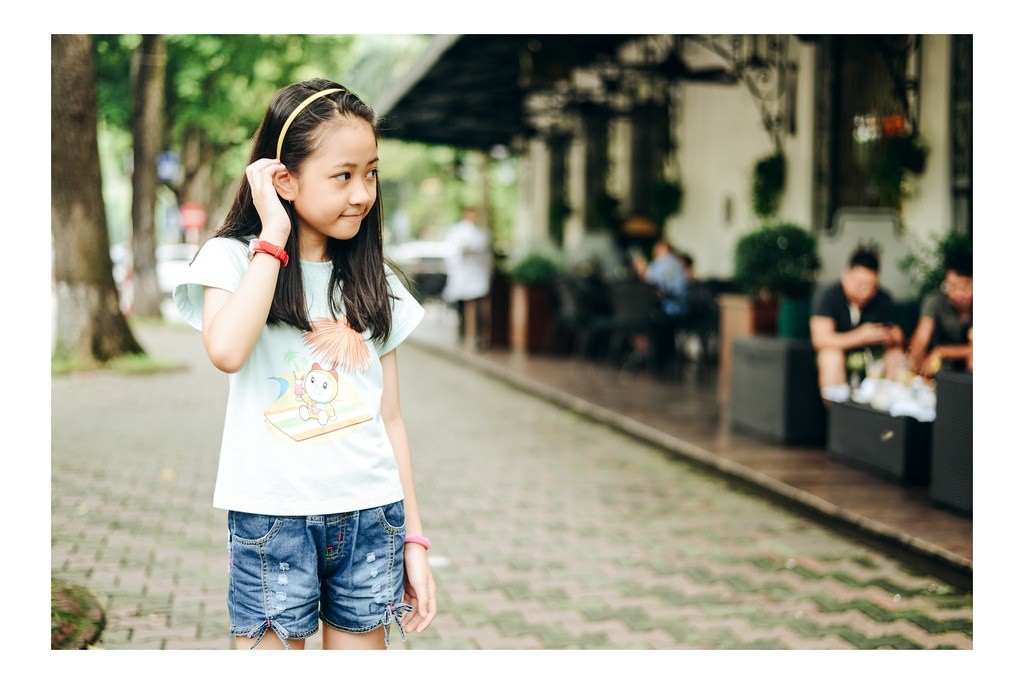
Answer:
[807,247,908,402]
[626,240,692,354]
[909,250,973,385]
[444,205,491,340]
[169,76,439,650]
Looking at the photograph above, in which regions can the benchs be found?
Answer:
[726,332,829,446]
[925,368,973,517]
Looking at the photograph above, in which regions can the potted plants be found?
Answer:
[713,226,819,406]
[507,255,563,354]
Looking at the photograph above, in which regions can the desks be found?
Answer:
[826,399,928,485]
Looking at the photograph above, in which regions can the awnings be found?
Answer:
[369,34,643,156]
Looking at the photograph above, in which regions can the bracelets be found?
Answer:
[404,535,430,550]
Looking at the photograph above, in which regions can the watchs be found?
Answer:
[247,238,289,267]
